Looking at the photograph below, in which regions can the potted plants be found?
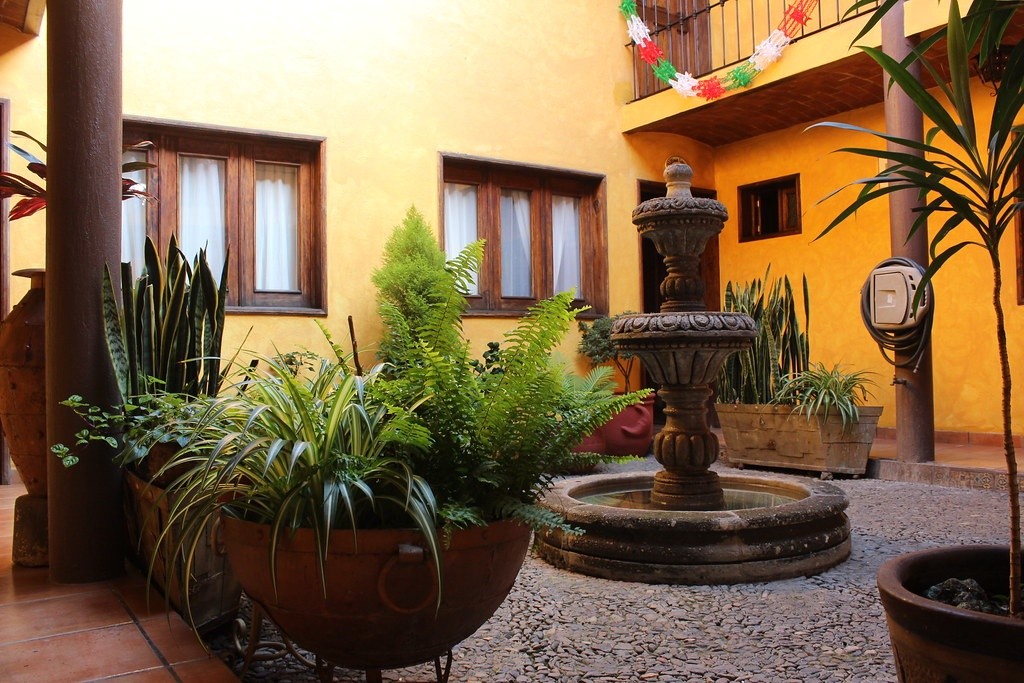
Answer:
[131,202,653,671]
[716,260,886,478]
[576,309,657,458]
[799,0,1024,683]
[101,227,259,638]
